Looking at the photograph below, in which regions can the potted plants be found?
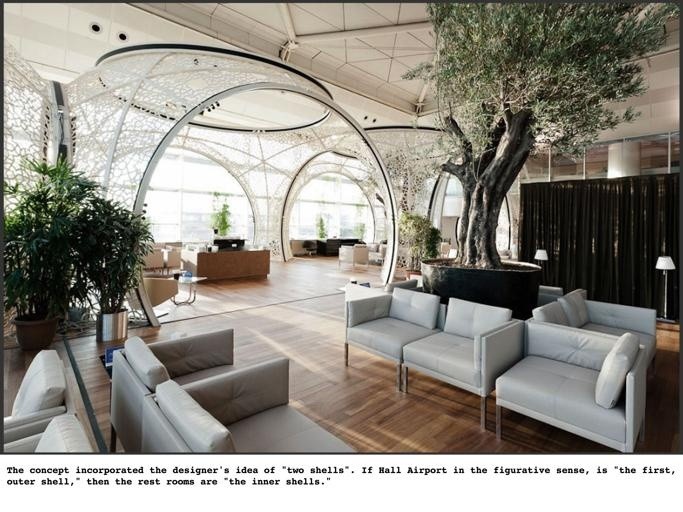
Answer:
[399,211,431,280]
[401,2,680,321]
[68,198,156,345]
[3,156,107,350]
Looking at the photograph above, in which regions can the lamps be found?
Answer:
[655,255,677,321]
[533,249,549,266]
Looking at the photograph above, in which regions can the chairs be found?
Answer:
[126,276,176,322]
[161,245,181,275]
[368,245,388,266]
[338,243,369,269]
[292,240,309,256]
[437,242,452,258]
[302,241,317,258]
[141,248,164,276]
[3,274,656,453]
